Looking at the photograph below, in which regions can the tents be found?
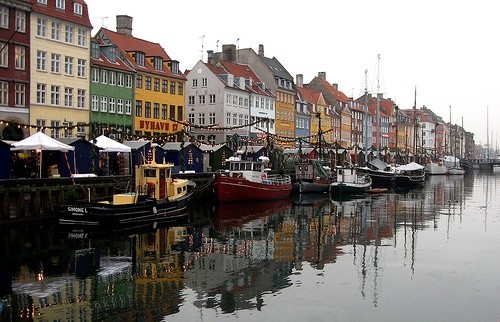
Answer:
[88,135,132,175]
[38,137,105,178]
[11,131,76,178]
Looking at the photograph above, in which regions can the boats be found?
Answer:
[214,140,292,201]
[58,152,198,235]
[300,158,469,197]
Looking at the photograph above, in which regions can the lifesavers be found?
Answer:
[261,174,266,180]
[144,182,155,193]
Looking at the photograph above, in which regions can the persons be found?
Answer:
[27,152,37,174]
[111,152,121,175]
